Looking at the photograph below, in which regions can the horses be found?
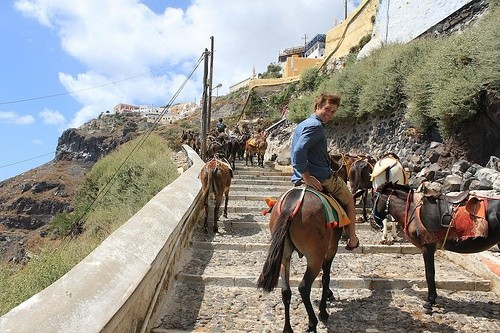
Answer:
[200,152,232,235]
[179,130,268,168]
[257,154,347,333]
[348,156,376,222]
[370,180,500,308]
[372,153,405,242]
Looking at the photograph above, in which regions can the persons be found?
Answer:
[217,118,227,133]
[290,91,359,251]
